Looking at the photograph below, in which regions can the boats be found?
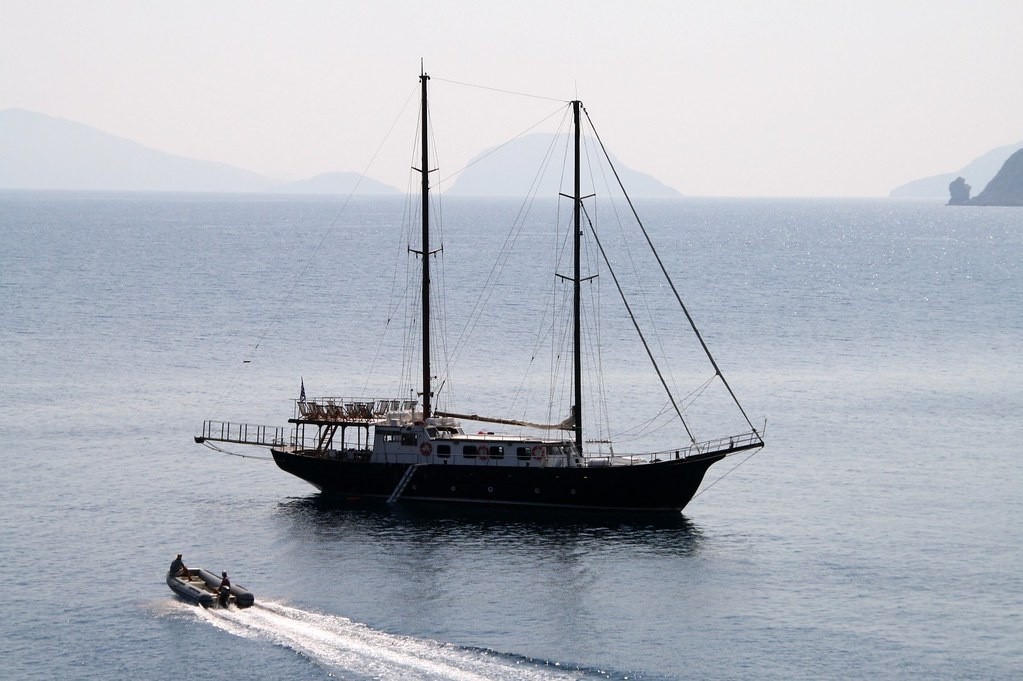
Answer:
[166,567,255,609]
[193,53,767,519]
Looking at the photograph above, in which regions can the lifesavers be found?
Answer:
[420,441,433,457]
[531,445,545,460]
[477,445,489,461]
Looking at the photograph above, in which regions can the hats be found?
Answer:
[177,553,182,558]
[222,570,227,574]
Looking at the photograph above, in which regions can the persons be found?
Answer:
[169,553,194,582]
[213,570,231,595]
